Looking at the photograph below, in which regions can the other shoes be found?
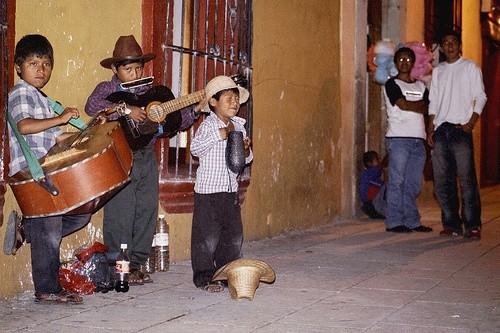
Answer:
[439,229,463,236]
[412,225,433,232]
[466,228,482,240]
[386,224,412,233]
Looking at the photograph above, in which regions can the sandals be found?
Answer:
[202,281,225,292]
[3,209,25,254]
[126,273,144,285]
[34,288,86,305]
[138,271,153,283]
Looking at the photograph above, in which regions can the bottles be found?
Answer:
[115,244,130,293]
[141,234,155,274]
[156,215,170,271]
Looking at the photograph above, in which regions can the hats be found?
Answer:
[99,35,156,68]
[211,260,276,303]
[200,76,250,112]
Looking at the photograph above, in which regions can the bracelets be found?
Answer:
[467,124,473,129]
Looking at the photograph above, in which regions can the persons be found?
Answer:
[383,47,432,232]
[190,75,255,293]
[84,35,209,285]
[3,35,92,305]
[426,30,488,239]
[363,159,425,219]
[359,151,389,202]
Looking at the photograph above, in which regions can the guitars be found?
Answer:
[8,101,133,218]
[104,73,248,151]
